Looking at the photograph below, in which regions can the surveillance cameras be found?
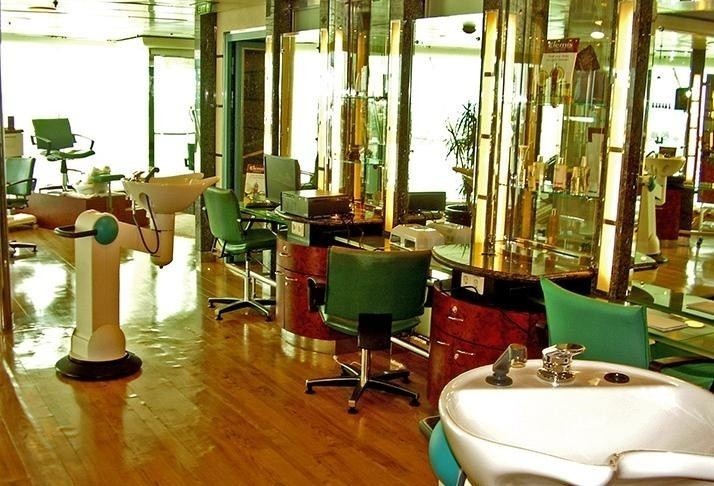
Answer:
[462,23,477,35]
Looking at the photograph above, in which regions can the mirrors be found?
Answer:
[277,1,713,368]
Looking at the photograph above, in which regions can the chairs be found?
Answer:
[207,154,713,415]
[4,116,97,262]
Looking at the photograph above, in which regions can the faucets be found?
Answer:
[491,344,526,380]
[645,150,654,157]
[142,167,160,182]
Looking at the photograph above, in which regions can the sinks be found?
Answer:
[646,153,685,177]
[121,172,220,212]
[437,358,714,486]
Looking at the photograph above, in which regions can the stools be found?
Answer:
[94,173,125,216]
